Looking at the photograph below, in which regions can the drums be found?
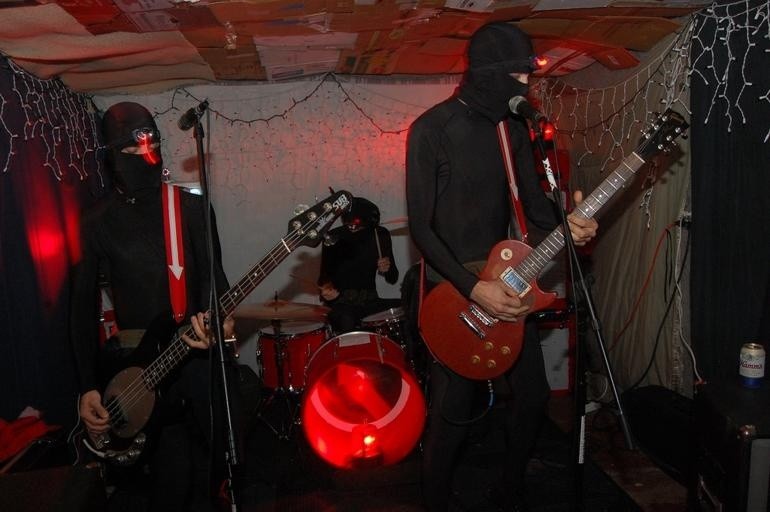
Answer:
[257,319,332,392]
[301,332,427,470]
[362,308,407,351]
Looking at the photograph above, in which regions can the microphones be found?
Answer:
[508,94,545,130]
[176,99,208,129]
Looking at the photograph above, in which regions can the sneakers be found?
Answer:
[420,474,467,512]
[482,470,531,511]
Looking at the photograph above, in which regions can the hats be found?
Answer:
[467,22,534,75]
[341,197,380,227]
[100,101,161,149]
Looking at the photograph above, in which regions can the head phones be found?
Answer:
[364,201,381,227]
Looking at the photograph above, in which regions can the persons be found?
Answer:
[70,102,235,512]
[405,21,598,511]
[318,198,399,333]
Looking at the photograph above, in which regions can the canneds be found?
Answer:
[739,342,766,390]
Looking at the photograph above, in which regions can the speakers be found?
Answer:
[689,376,770,510]
[626,383,694,483]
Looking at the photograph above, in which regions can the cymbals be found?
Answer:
[231,301,330,320]
[380,217,408,224]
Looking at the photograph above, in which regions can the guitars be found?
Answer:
[419,108,691,379]
[77,188,354,462]
